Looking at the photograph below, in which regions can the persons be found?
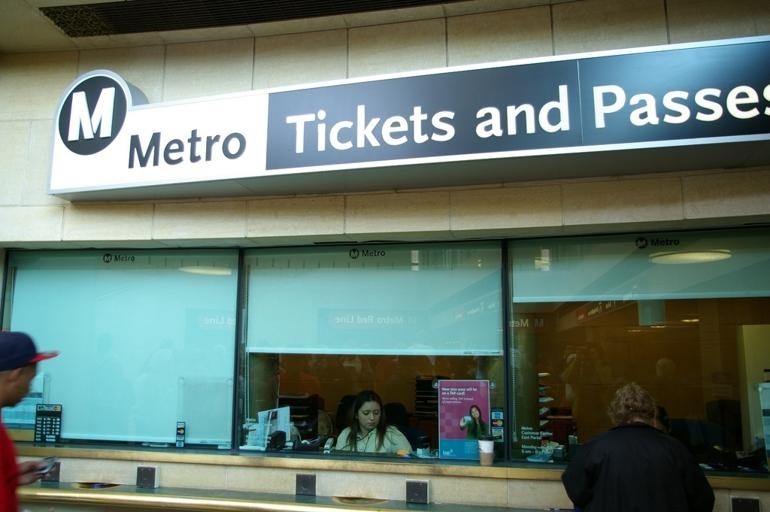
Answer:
[0,331,58,512]
[312,391,431,455]
[655,406,704,465]
[560,381,714,512]
[458,405,489,440]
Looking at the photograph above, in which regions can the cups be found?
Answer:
[478,436,496,466]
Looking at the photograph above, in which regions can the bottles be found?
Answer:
[416,436,431,458]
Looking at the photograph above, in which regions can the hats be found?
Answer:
[0,331,61,371]
